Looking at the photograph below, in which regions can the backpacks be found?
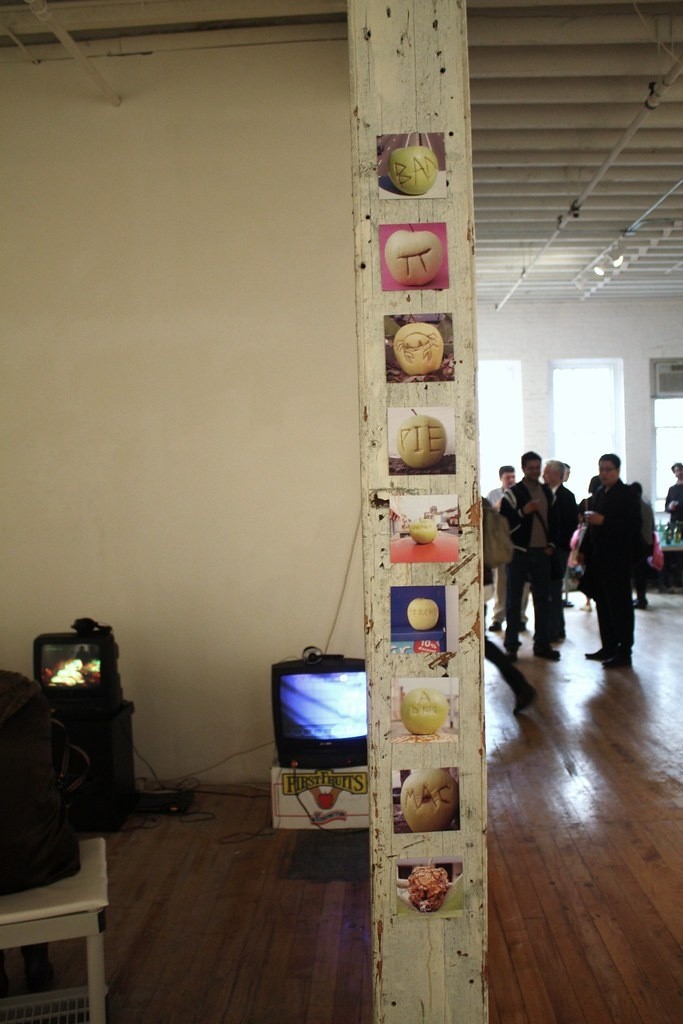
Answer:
[483,507,513,567]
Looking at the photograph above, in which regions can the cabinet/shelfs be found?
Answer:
[52,702,138,834]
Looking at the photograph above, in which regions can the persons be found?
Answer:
[0,671,82,996]
[482,450,656,717]
[665,463,683,528]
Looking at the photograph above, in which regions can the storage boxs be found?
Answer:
[269,759,370,830]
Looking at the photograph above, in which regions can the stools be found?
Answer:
[0,834,110,1024]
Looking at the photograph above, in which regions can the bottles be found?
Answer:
[674,521,680,544]
[666,522,671,544]
[658,520,664,545]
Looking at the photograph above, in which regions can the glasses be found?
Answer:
[599,467,616,473]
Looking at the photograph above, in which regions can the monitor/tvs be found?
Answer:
[271,657,369,769]
[32,631,118,704]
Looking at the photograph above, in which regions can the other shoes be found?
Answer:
[25,960,54,993]
[585,647,611,659]
[505,654,517,662]
[489,622,501,631]
[519,622,526,631]
[548,628,565,640]
[602,654,631,667]
[533,647,559,659]
[513,683,536,715]
[631,598,648,608]
[580,604,592,611]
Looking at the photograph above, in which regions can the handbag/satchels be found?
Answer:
[549,551,564,582]
[648,531,663,571]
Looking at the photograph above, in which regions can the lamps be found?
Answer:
[593,258,608,275]
[606,240,626,267]
